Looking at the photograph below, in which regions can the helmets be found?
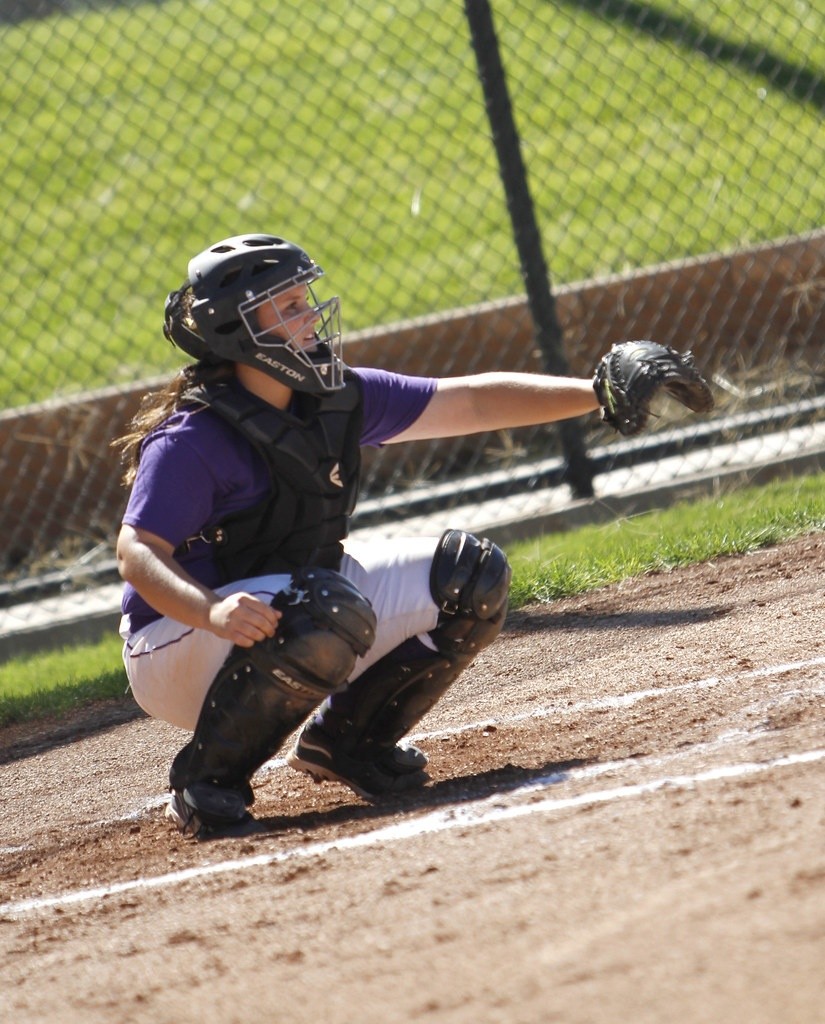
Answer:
[163,233,348,397]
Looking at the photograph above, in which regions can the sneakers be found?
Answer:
[287,718,421,802]
[164,786,268,842]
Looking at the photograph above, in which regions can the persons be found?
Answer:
[117,234,712,841]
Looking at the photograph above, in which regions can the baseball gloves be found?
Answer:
[593,340,715,438]
